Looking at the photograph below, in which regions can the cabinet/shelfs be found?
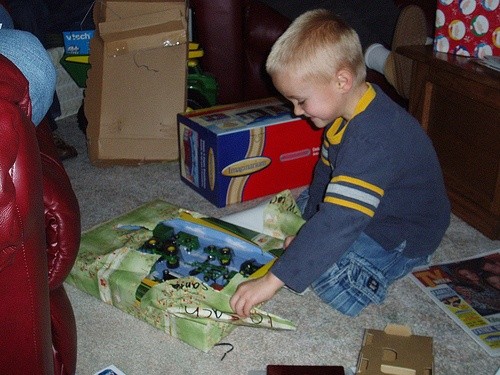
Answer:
[395,44,500,241]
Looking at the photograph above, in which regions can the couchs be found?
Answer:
[0,53,81,375]
[191,0,436,103]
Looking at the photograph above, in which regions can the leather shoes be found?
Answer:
[52,132,79,160]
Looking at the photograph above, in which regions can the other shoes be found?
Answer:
[383,6,427,100]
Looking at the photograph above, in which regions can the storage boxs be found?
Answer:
[83,0,189,168]
[177,97,326,208]
[433,0,500,58]
[64,30,95,54]
[63,199,287,353]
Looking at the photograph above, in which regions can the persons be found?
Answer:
[0,6,76,158]
[229,10,452,317]
